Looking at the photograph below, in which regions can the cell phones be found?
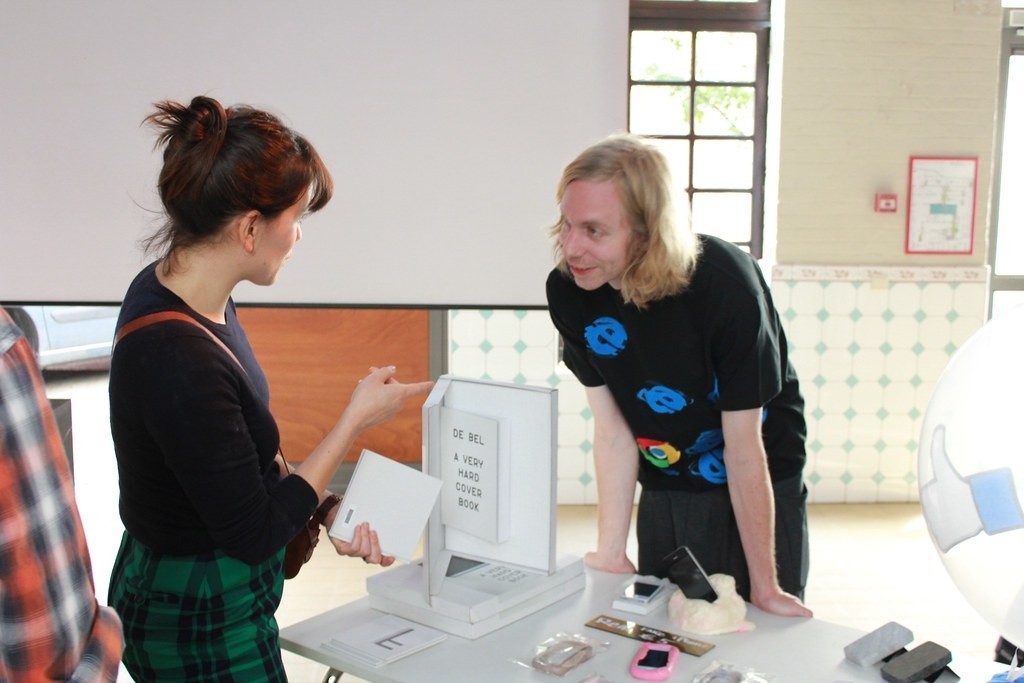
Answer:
[619,577,663,606]
[662,545,719,605]
[630,642,679,681]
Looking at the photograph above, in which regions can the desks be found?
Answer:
[276,564,1024,683]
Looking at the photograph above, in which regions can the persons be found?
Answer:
[545,133,814,619]
[108,94,434,683]
[0,300,126,683]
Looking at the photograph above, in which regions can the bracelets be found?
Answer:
[314,495,341,524]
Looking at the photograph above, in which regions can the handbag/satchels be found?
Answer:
[283,519,320,581]
